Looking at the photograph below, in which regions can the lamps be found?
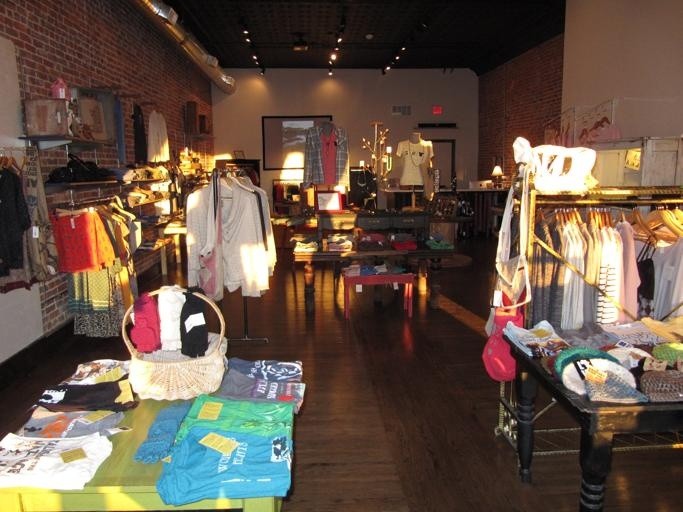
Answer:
[323,36,344,77]
[243,26,268,77]
[380,45,407,74]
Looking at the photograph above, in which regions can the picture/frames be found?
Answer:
[261,114,335,172]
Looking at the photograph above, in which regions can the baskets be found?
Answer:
[121,287,228,400]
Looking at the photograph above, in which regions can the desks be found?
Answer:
[0,360,305,511]
[510,320,681,510]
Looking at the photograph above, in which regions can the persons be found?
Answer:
[303,121,350,187]
[396,131,434,190]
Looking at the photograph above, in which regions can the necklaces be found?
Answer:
[409,141,424,167]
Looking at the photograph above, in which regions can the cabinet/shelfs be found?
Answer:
[25,131,129,339]
[124,159,169,298]
[492,190,505,238]
[288,185,464,321]
[171,166,207,263]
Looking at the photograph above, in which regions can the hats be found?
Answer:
[545,338,683,404]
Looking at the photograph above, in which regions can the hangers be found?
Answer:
[529,200,682,250]
